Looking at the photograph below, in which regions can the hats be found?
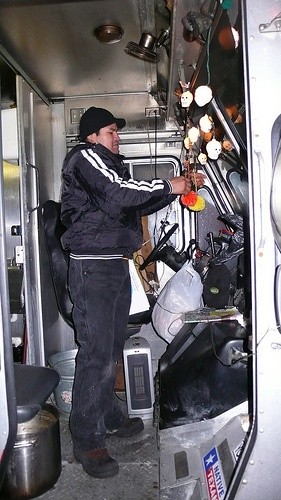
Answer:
[79,106,127,142]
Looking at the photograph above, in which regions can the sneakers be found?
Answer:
[74,438,119,478]
[105,413,144,438]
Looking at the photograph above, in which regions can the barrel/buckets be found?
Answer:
[49,349,78,414]
[4,403,61,500]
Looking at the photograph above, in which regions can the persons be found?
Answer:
[59,107,207,478]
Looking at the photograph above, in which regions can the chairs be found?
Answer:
[13,358,60,423]
[40,201,156,341]
